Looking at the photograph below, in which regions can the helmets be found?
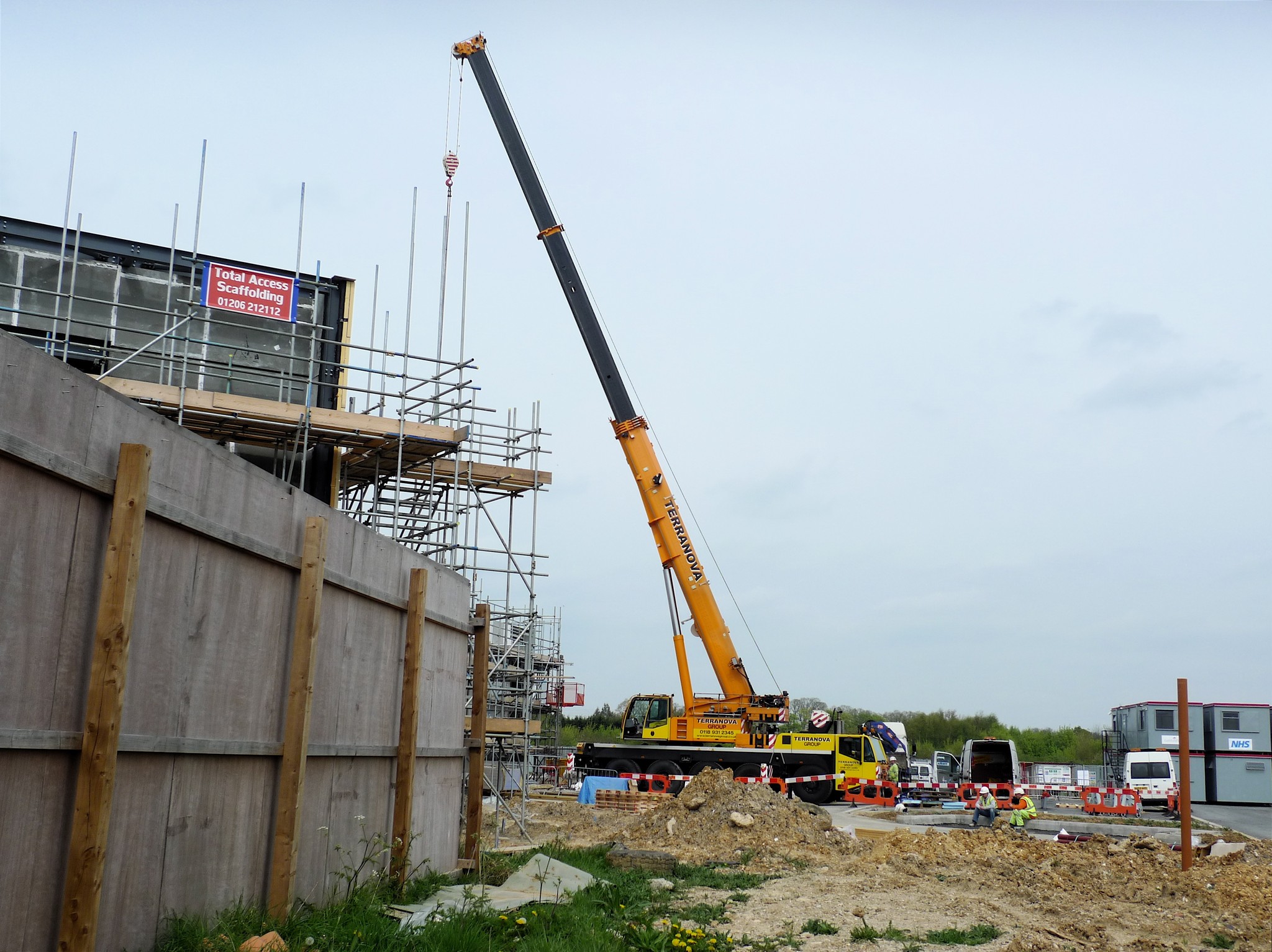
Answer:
[890,756,896,761]
[979,786,989,794]
[1014,788,1024,794]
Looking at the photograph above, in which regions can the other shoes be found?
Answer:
[1014,825,1024,829]
[969,822,977,826]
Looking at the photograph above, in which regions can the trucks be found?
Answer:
[856,721,963,804]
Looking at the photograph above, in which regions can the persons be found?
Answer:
[1007,787,1038,828]
[888,756,898,786]
[1074,782,1078,797]
[969,787,1000,828]
[849,748,861,759]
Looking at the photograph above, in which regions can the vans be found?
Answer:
[1123,747,1179,812]
[957,738,1021,811]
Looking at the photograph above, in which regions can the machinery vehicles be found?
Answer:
[443,32,899,808]
